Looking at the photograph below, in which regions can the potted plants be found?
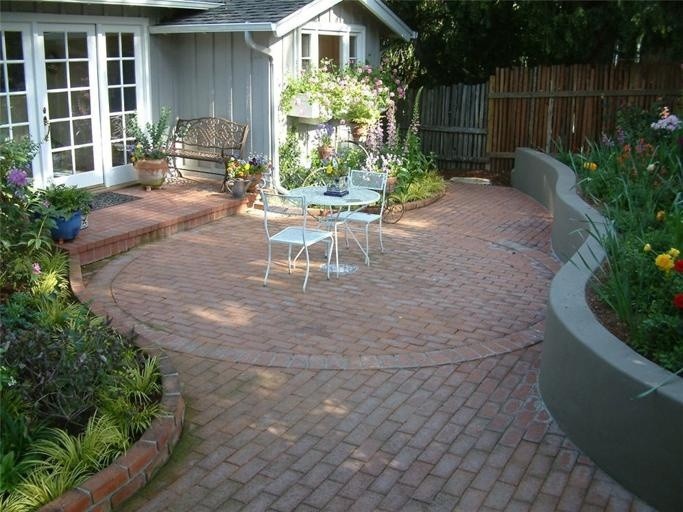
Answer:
[78,189,94,230]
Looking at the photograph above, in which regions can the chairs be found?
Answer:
[327,170,388,264]
[260,192,333,292]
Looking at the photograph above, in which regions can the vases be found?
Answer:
[289,94,318,118]
[50,211,83,241]
[236,173,261,192]
[136,159,169,191]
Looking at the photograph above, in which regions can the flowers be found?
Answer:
[36,182,93,221]
[309,73,348,115]
[226,155,251,181]
[113,105,191,159]
[244,151,275,178]
[298,111,331,126]
[278,68,329,105]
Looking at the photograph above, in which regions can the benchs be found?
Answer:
[165,118,249,192]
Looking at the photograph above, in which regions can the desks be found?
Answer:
[288,187,383,280]
